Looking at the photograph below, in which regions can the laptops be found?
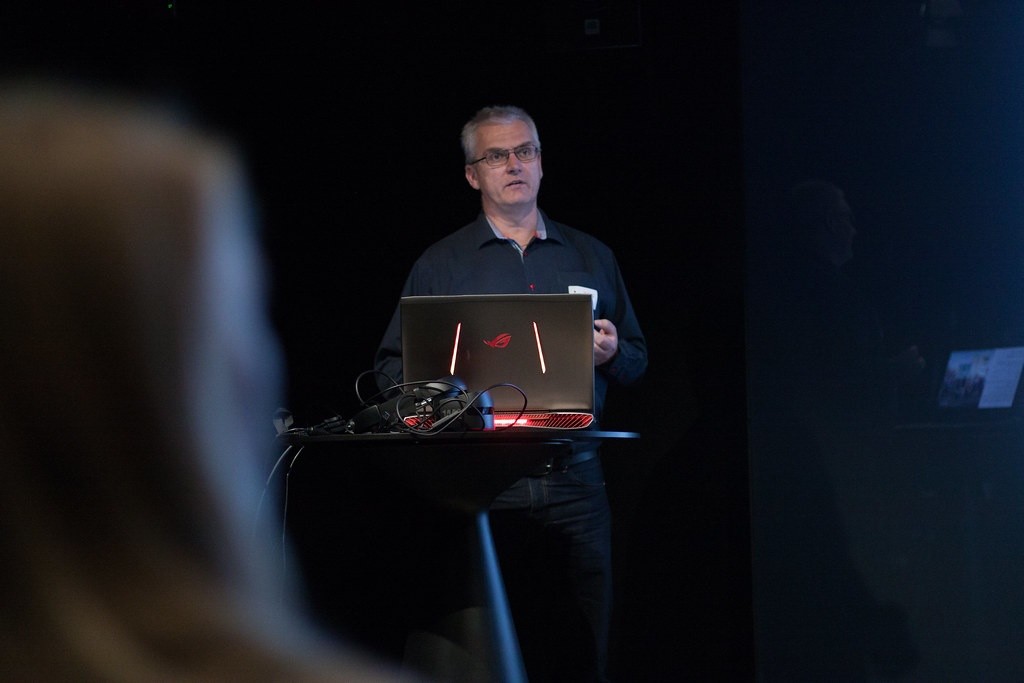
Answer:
[914,344,1023,422]
[401,294,594,414]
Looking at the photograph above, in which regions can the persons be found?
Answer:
[374,106,647,683]
[0,84,413,683]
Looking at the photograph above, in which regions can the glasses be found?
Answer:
[471,145,541,166]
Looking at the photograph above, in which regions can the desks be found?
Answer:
[281,424,642,682]
